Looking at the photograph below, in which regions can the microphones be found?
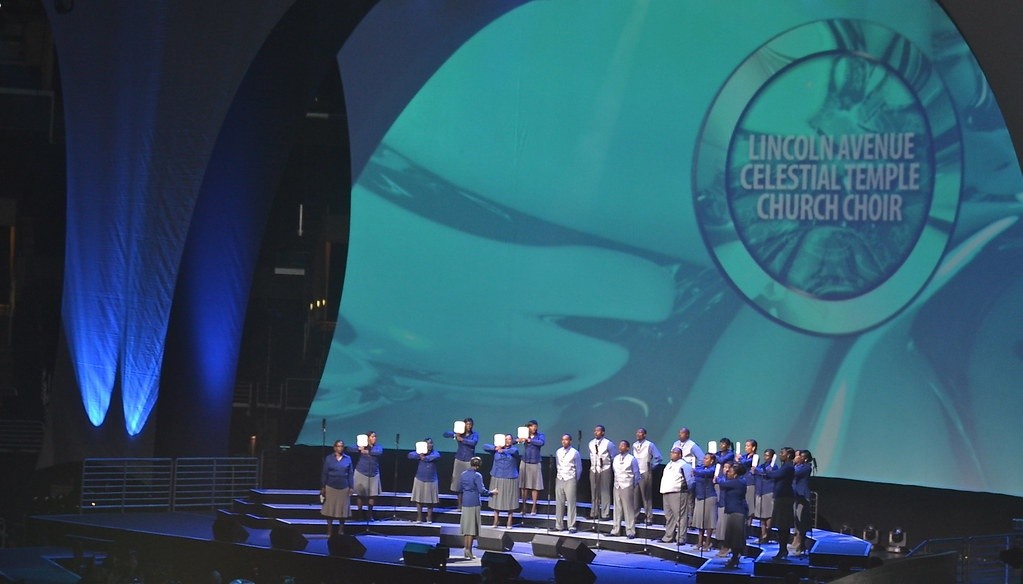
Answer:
[812,457,817,471]
[322,419,326,428]
[578,430,582,438]
[595,444,598,452]
[524,440,528,447]
[396,434,399,443]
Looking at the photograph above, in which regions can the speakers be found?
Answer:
[477,529,514,552]
[213,519,249,544]
[440,526,466,548]
[481,551,523,578]
[531,535,596,584]
[327,534,367,557]
[269,527,309,550]
[403,542,440,568]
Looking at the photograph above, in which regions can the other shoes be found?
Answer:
[646,522,652,526]
[676,542,685,546]
[570,529,577,533]
[657,539,673,543]
[634,520,640,524]
[548,527,559,531]
[603,531,615,536]
[587,516,599,520]
[628,534,634,539]
[600,517,610,521]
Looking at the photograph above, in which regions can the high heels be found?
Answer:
[426,516,432,524]
[521,509,527,514]
[531,509,538,515]
[417,520,421,523]
[492,521,500,528]
[507,522,512,529]
[693,541,712,552]
[724,531,807,569]
[463,548,476,560]
[716,548,731,557]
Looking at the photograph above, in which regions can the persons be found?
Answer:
[587,425,618,521]
[457,457,498,559]
[657,446,695,546]
[320,440,354,538]
[670,427,705,531]
[482,434,520,530]
[692,438,817,570]
[632,427,662,526]
[352,431,385,521]
[514,419,545,515]
[550,434,583,532]
[443,417,478,511]
[606,440,640,539]
[408,438,441,525]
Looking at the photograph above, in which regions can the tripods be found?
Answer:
[587,452,707,577]
[510,446,535,528]
[358,443,412,535]
